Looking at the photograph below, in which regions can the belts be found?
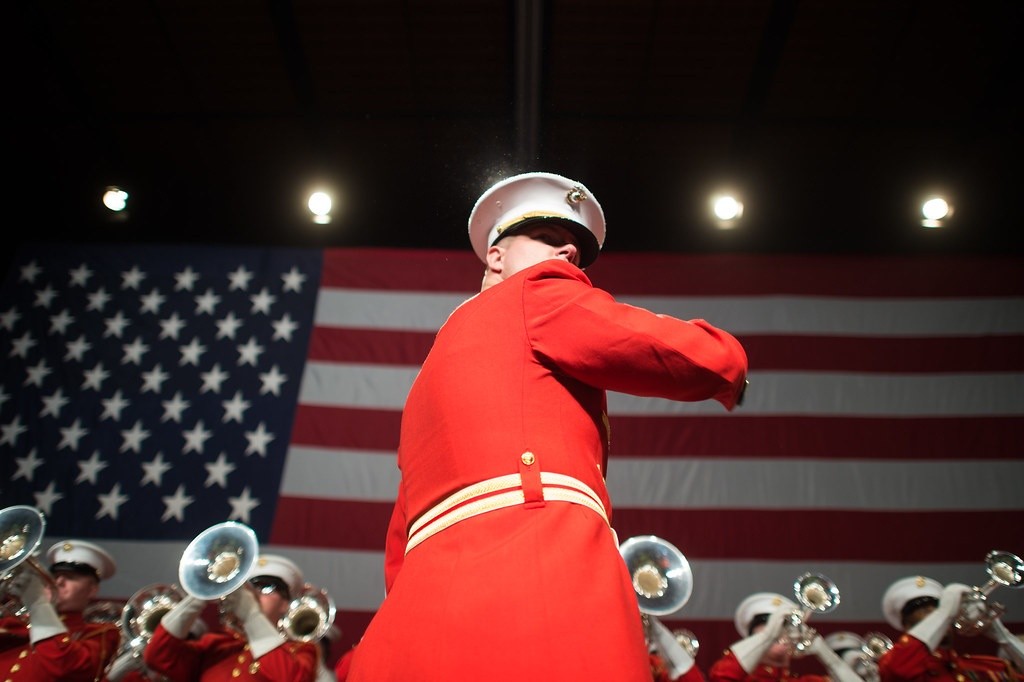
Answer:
[405,472,619,551]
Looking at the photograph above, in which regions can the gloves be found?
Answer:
[844,651,868,675]
[226,586,286,661]
[730,608,795,675]
[908,583,975,652]
[650,615,696,681]
[7,570,68,646]
[160,595,206,638]
[984,611,1023,670]
[798,635,865,681]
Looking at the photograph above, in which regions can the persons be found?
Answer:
[0,541,353,682]
[347,172,748,682]
[641,575,1024,682]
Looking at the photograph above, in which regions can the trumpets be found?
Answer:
[118,521,344,682]
[670,549,1024,682]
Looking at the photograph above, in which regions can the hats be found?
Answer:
[250,555,304,603]
[735,592,799,639]
[827,631,866,652]
[468,172,607,264]
[323,626,340,644]
[47,540,116,584]
[883,577,945,631]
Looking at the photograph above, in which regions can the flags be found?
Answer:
[0,249,1024,674]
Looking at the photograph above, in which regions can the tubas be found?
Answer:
[617,534,695,656]
[0,505,58,630]
[177,521,261,603]
